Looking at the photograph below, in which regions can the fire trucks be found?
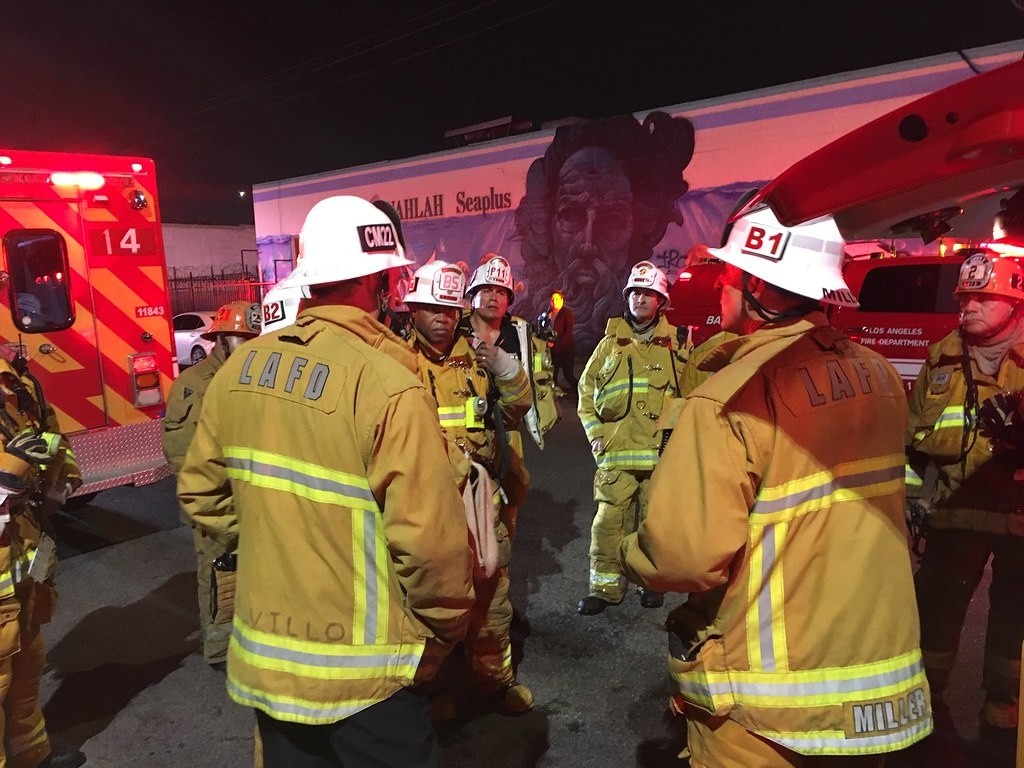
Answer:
[660,56,1024,406]
[1,147,181,511]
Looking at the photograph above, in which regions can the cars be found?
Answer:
[172,311,219,366]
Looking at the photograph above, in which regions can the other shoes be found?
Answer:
[420,688,458,721]
[37,747,86,768]
[640,590,663,608]
[497,681,534,711]
[981,697,1020,729]
[577,597,608,615]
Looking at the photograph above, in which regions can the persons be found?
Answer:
[162,299,262,671]
[400,260,534,711]
[459,256,558,542]
[904,256,1024,768]
[576,261,693,618]
[176,196,475,768]
[0,353,86,768]
[615,203,933,768]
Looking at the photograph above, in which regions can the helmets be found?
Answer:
[199,301,261,341]
[403,260,465,308]
[622,261,671,310]
[707,204,859,306]
[953,253,1024,300]
[299,195,415,286]
[465,256,515,306]
[260,273,311,336]
